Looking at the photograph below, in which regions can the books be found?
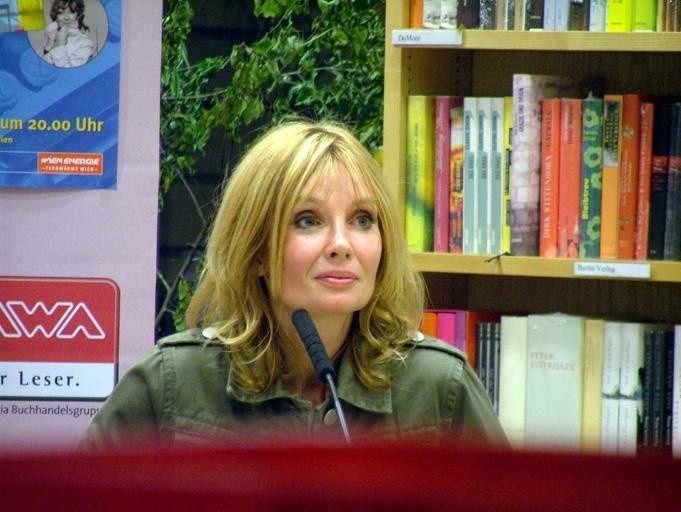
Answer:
[420,307,680,458]
[404,70,680,260]
[408,1,680,33]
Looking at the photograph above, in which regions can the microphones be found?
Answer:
[291,307,353,442]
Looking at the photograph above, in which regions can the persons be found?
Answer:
[43,0,94,69]
[74,117,511,448]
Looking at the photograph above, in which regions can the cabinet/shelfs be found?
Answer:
[382,3,681,455]
